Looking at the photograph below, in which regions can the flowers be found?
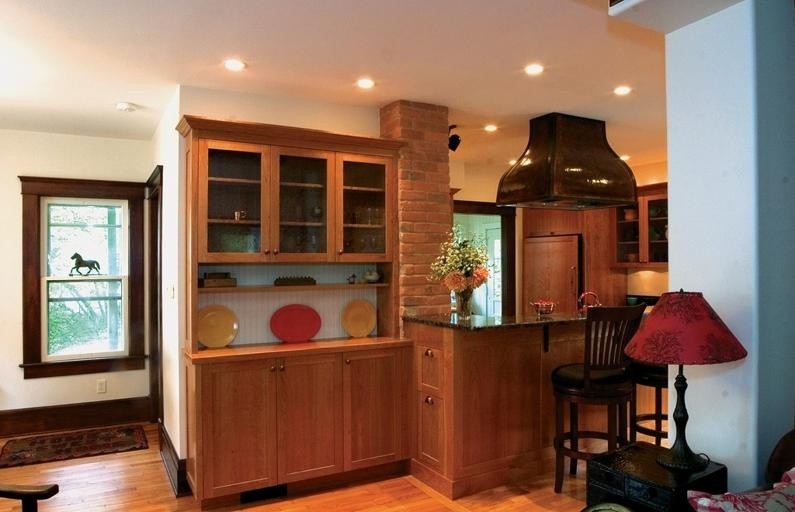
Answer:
[424,222,492,317]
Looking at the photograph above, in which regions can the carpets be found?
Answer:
[1,423,150,469]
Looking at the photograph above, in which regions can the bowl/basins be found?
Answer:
[536,317,554,322]
[534,302,555,315]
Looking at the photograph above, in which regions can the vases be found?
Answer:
[453,287,476,321]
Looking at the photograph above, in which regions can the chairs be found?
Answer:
[623,292,671,451]
[550,302,649,493]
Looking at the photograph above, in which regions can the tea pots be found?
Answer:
[577,291,602,315]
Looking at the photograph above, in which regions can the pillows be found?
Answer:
[687,467,794,512]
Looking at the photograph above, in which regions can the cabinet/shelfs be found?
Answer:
[414,343,448,470]
[185,354,345,501]
[182,131,335,265]
[345,348,413,472]
[334,147,399,265]
[585,441,730,512]
[611,180,667,271]
[518,204,628,314]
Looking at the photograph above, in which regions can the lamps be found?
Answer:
[448,123,463,152]
[623,288,749,472]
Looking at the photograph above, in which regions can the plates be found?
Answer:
[269,303,320,344]
[197,304,238,349]
[341,299,377,336]
